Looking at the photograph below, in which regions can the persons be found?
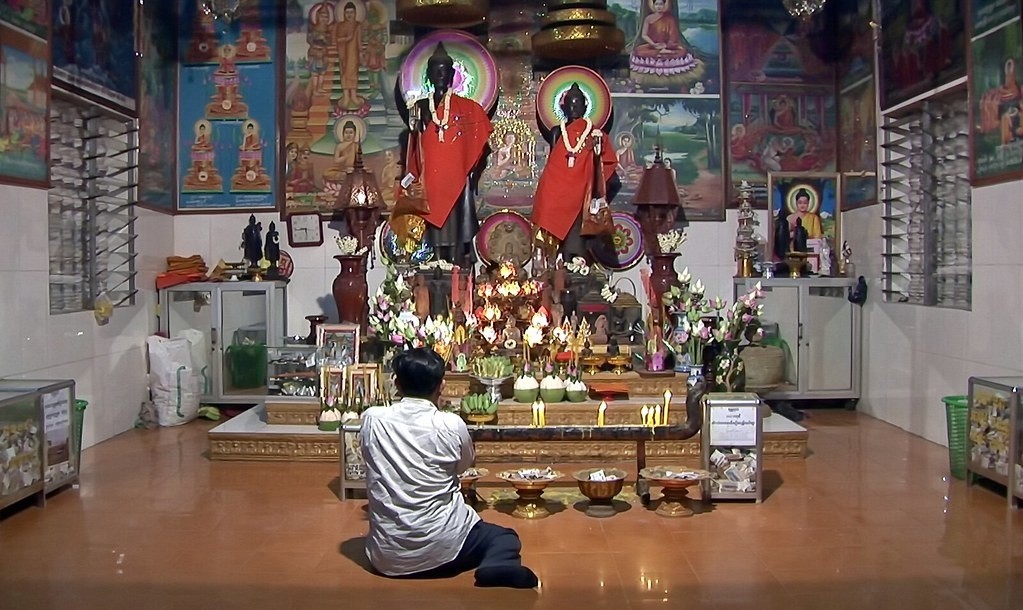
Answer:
[500,242,521,271]
[532,81,621,265]
[239,215,262,268]
[263,219,283,276]
[541,277,578,326]
[773,207,831,278]
[407,265,452,327]
[397,40,493,268]
[358,347,538,590]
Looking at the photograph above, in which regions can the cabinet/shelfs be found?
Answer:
[160,282,288,403]
[734,276,863,405]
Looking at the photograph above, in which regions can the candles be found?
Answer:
[655,404,662,425]
[640,405,648,424]
[663,390,672,424]
[532,401,538,425]
[538,400,546,426]
[647,406,655,424]
[597,400,607,426]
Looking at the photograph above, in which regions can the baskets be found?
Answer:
[941,394,970,480]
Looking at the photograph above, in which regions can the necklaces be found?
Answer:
[560,116,592,167]
[429,87,453,142]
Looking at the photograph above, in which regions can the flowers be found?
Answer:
[337,229,770,390]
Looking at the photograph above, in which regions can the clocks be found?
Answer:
[288,211,323,247]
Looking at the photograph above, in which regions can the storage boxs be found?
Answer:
[0,378,81,511]
[701,392,762,503]
[339,418,366,501]
[967,377,1023,506]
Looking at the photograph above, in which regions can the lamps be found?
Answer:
[332,132,388,249]
[632,125,682,228]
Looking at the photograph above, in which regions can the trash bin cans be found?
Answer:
[942,395,969,481]
[75,400,89,475]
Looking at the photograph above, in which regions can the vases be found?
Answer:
[332,252,784,403]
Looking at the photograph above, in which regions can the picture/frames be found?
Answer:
[0,0,1023,275]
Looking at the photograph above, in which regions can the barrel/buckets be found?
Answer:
[230,345,267,388]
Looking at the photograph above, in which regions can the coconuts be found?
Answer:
[514,375,587,403]
[319,406,362,430]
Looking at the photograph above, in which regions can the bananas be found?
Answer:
[459,393,499,415]
[471,356,514,378]
